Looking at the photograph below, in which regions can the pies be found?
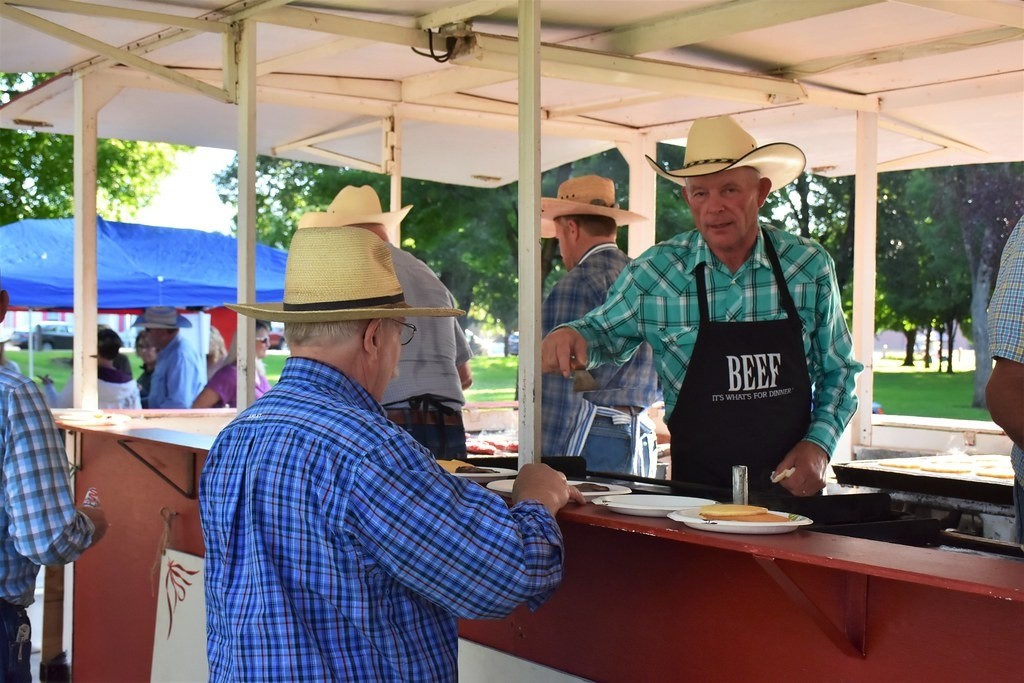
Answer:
[437,458,475,473]
[699,503,789,522]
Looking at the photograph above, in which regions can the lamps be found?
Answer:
[445,20,807,104]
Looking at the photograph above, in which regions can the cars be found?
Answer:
[267,329,286,350]
[11,322,73,350]
[466,329,487,355]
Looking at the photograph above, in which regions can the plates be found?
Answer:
[60,414,131,425]
[486,480,632,503]
[667,510,813,534]
[592,495,716,516]
[450,467,518,482]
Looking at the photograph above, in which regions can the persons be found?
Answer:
[35,307,273,409]
[0,290,107,683]
[199,226,586,683]
[541,115,864,495]
[986,214,1024,545]
[298,185,474,459]
[541,176,658,479]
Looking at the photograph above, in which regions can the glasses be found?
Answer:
[256,337,267,343]
[145,328,151,333]
[392,318,416,345]
[137,343,155,348]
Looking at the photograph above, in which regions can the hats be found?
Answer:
[540,175,650,238]
[223,227,466,322]
[131,305,192,328]
[297,185,413,229]
[645,114,807,194]
[0,322,11,343]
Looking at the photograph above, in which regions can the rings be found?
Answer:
[803,490,806,494]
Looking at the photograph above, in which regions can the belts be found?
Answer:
[386,410,458,426]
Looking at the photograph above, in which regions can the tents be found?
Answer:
[0,213,289,378]
